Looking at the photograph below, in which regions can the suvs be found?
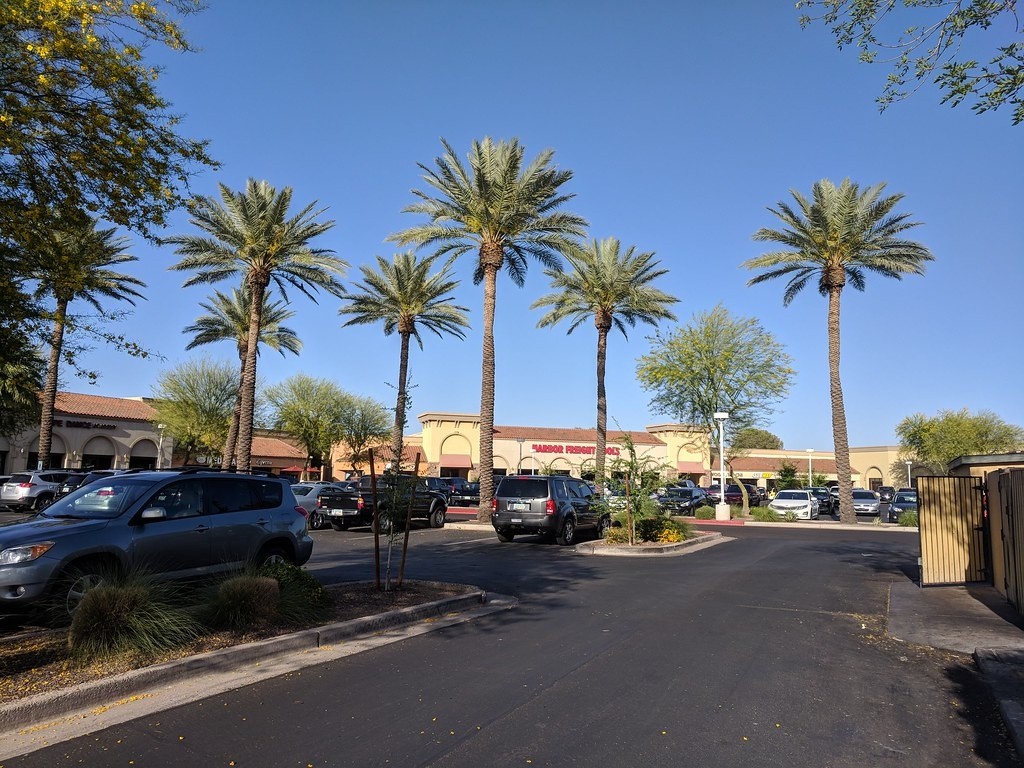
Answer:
[0,466,315,623]
[490,472,612,545]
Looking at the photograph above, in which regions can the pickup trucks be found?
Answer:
[316,473,450,535]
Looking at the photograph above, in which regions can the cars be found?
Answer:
[588,481,729,516]
[805,484,840,513]
[724,483,760,507]
[285,473,497,530]
[887,488,919,522]
[878,486,897,503]
[765,489,820,520]
[754,486,768,501]
[0,468,191,518]
[851,490,882,516]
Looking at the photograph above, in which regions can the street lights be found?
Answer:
[516,438,525,476]
[805,448,815,487]
[905,461,914,488]
[710,411,733,520]
[153,422,169,470]
